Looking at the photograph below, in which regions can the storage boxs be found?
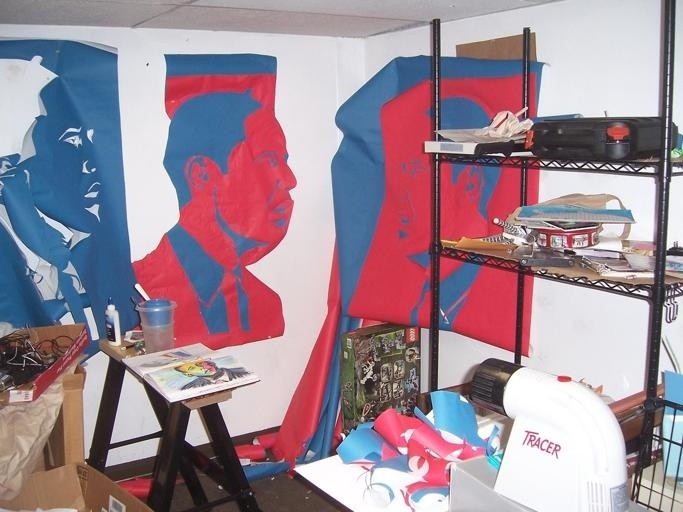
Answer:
[341,324,423,432]
[48,363,84,467]
[0,459,158,511]
[0,320,90,405]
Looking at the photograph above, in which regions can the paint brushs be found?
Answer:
[121,340,135,352]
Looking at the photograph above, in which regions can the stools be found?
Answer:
[87,338,262,512]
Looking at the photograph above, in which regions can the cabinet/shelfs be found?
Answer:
[429,0,682,479]
